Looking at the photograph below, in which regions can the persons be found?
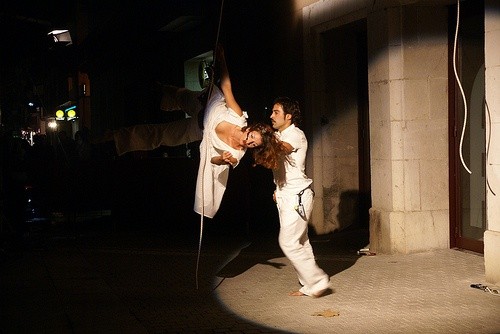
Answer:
[193,40,291,219]
[269,97,332,298]
